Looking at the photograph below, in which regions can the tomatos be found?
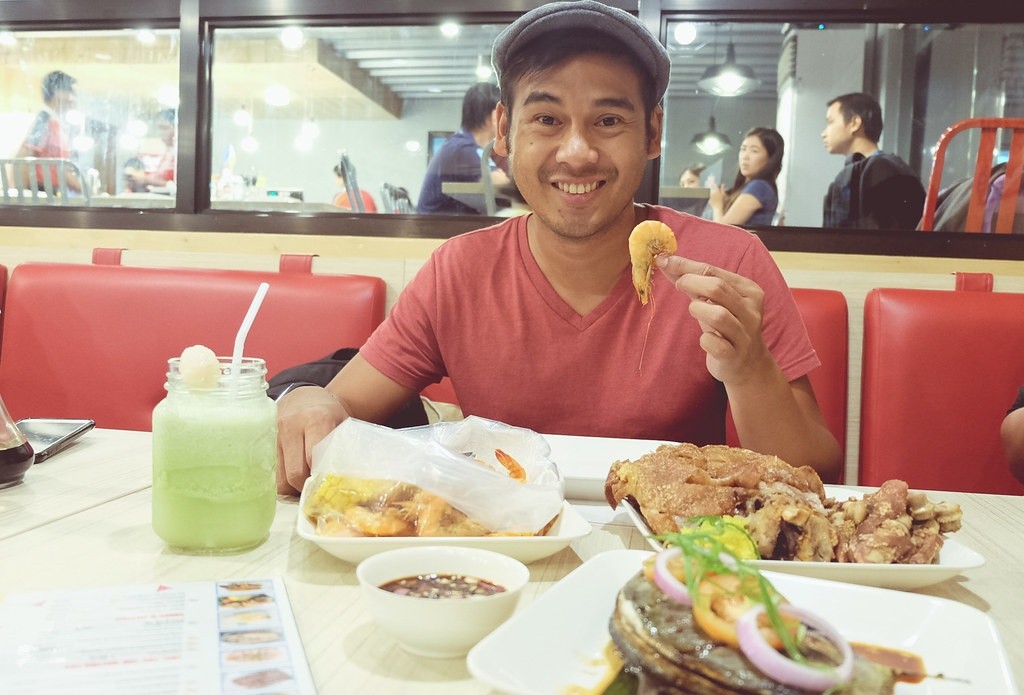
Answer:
[690,573,800,652]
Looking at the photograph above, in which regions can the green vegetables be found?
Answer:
[641,516,807,665]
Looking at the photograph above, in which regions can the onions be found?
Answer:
[654,546,855,691]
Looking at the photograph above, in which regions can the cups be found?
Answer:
[152,356,278,556]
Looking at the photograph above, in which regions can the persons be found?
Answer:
[335,164,379,213]
[272,0,841,496]
[823,93,926,230]
[6,70,177,197]
[1000,387,1024,485]
[679,127,784,227]
[416,84,530,216]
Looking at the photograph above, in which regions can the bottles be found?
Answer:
[0,394,32,492]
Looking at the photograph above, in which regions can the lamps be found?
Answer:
[688,22,733,155]
[699,22,762,96]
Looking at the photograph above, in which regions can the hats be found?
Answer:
[491,0,669,105]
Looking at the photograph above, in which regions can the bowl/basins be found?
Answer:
[354,544,530,661]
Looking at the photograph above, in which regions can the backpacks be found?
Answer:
[266,348,429,429]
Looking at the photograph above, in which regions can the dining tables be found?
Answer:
[0,431,1023,695]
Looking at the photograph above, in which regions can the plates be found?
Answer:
[299,483,594,574]
[468,547,1016,695]
[215,575,302,694]
[613,487,985,593]
[544,429,685,504]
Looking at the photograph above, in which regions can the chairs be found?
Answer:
[0,157,88,199]
[921,117,1024,233]
[339,155,413,215]
[0,248,1024,496]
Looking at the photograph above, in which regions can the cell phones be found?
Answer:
[16,418,95,464]
